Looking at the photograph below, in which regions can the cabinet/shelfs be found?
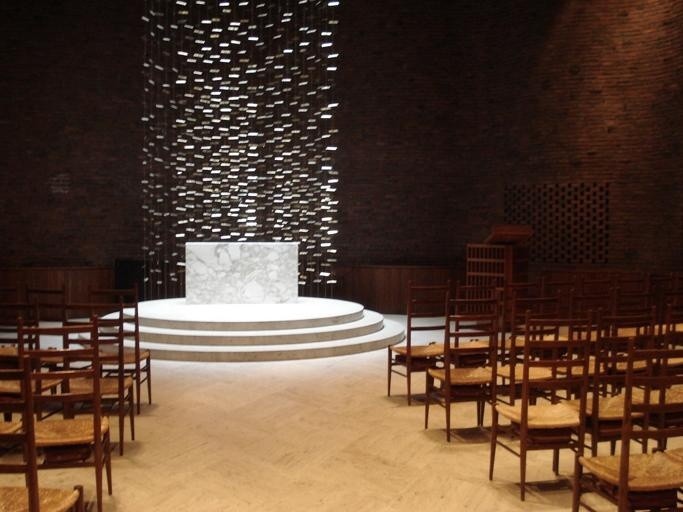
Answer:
[464,241,531,318]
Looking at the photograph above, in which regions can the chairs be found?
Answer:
[2,281,154,512]
[386,264,683,512]
